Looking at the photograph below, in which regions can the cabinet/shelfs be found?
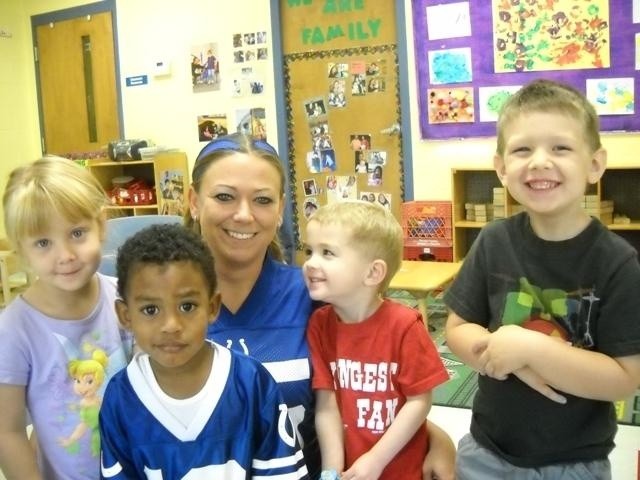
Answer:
[450,166,640,261]
[89,152,190,219]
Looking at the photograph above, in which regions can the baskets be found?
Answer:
[401,245,453,262]
[399,199,453,249]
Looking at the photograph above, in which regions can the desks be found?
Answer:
[389,260,461,332]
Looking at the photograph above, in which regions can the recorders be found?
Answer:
[107,140,147,162]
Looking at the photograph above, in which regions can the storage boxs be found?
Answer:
[404,247,453,262]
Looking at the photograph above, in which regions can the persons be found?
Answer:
[305,62,389,219]
[0,155,143,480]
[192,49,218,87]
[98,223,311,480]
[444,80,640,480]
[182,133,457,480]
[305,199,450,480]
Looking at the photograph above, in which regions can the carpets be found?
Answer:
[387,289,640,426]
